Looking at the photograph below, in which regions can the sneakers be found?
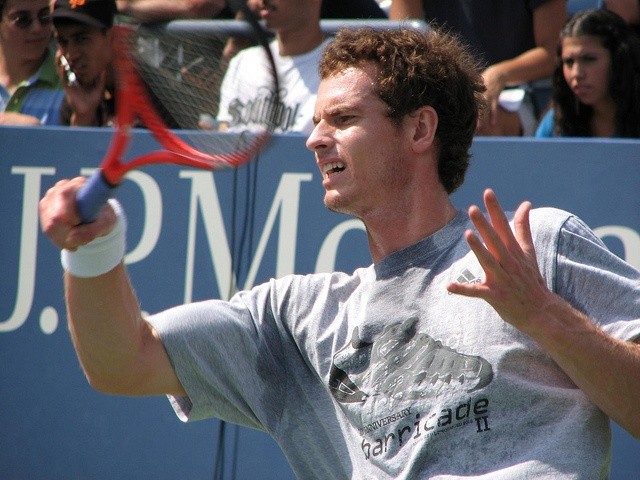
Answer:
[328,317,495,404]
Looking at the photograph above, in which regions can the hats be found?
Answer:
[52,0,118,32]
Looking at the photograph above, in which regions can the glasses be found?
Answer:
[14,8,52,29]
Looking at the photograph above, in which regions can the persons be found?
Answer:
[49,0,179,130]
[35,27,640,479]
[198,0,341,135]
[533,6,639,139]
[111,0,227,26]
[217,0,266,63]
[0,0,74,128]
[565,0,639,25]
[387,0,568,137]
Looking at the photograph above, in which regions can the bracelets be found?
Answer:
[59,196,128,281]
